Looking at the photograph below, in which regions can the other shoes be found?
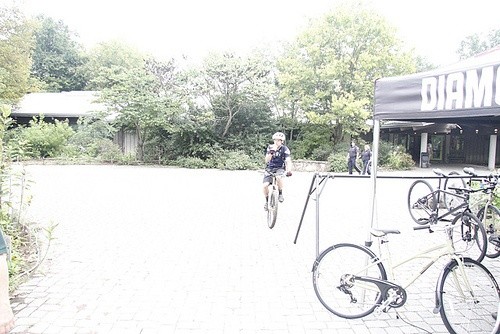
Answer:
[264,202,268,210]
[279,195,283,201]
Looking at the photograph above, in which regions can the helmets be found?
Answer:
[272,132,285,143]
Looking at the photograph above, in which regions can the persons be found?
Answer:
[263,132,293,211]
[347,141,362,176]
[358,145,372,176]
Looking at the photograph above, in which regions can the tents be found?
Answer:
[358,45,500,317]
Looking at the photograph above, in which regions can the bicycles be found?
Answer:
[264,168,291,229]
[312,216,500,334]
[407,167,500,267]
